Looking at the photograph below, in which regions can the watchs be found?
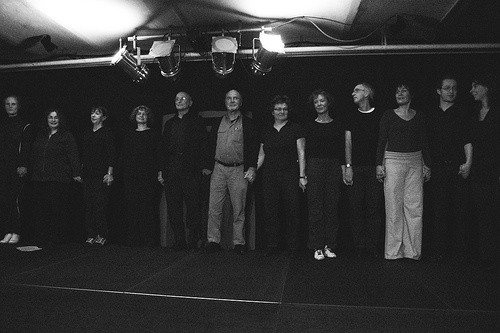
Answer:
[300,176,308,179]
[106,172,113,175]
[345,164,351,167]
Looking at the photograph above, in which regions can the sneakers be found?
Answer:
[94,235,108,247]
[9,233,20,244]
[314,249,324,260]
[84,237,96,248]
[0,232,12,243]
[324,248,336,257]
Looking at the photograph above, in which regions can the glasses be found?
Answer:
[274,107,288,112]
[442,85,458,91]
[352,88,365,94]
[175,97,189,102]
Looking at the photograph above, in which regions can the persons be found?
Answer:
[459,71,500,260]
[345,83,386,257]
[104,103,166,247]
[199,88,256,253]
[376,80,432,265]
[78,103,116,246]
[0,93,31,244]
[16,108,82,245]
[298,89,354,260]
[243,95,308,251]
[158,92,213,253]
[423,74,475,245]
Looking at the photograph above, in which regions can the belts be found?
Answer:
[214,158,244,167]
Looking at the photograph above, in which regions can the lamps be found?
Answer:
[211,31,238,75]
[109,35,151,83]
[149,36,182,78]
[250,26,286,76]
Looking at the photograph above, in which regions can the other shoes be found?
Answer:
[266,244,303,258]
[233,244,248,254]
[172,239,197,253]
[200,242,222,254]
